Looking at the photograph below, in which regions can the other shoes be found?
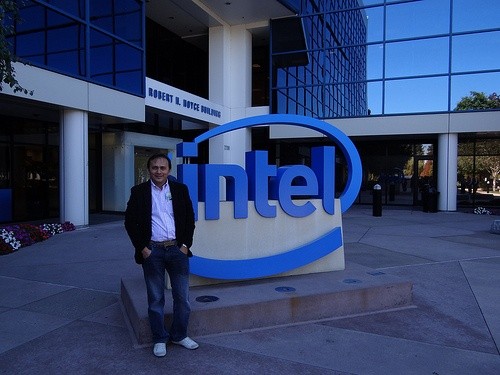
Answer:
[153,343,166,357]
[172,337,199,350]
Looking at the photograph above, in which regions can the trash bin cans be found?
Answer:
[424,190,440,213]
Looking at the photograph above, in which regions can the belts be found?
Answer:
[151,240,178,247]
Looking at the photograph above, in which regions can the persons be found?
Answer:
[124,155,200,357]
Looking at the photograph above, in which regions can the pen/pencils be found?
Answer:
[167,197,169,202]
